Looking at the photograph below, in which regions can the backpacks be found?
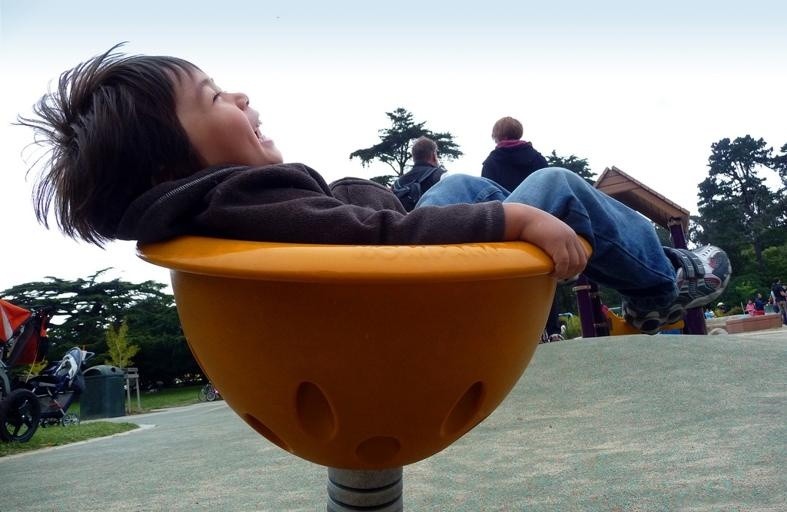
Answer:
[393,168,436,211]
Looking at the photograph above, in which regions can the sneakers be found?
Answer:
[622,243,733,336]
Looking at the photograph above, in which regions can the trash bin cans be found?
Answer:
[79,365,125,420]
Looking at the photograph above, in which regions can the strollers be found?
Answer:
[0,298,59,444]
[23,347,94,432]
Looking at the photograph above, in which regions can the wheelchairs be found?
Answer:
[198,381,215,402]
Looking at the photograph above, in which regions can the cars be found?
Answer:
[171,373,202,385]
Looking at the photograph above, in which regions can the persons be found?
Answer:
[481,118,569,343]
[15,38,733,333]
[390,139,447,212]
[704,308,718,317]
[745,279,787,331]
